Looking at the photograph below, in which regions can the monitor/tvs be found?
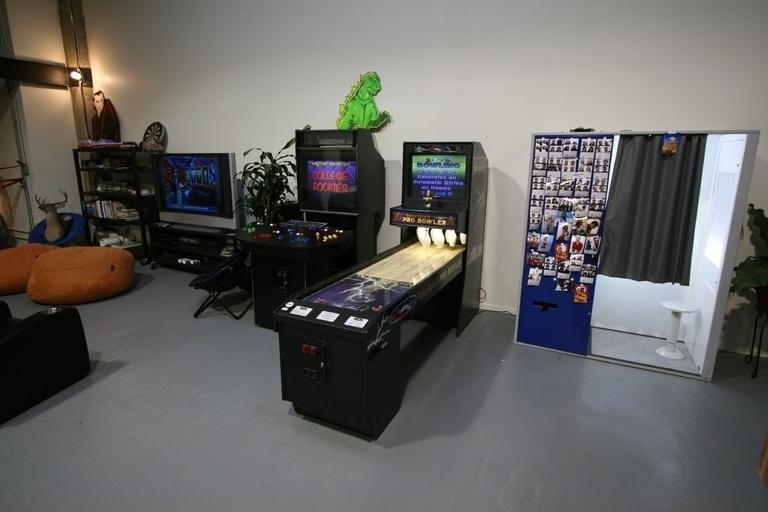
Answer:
[402,142,472,209]
[298,147,359,213]
[151,153,241,230]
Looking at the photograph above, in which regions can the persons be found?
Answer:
[525,139,609,305]
[91,89,121,143]
[661,135,678,154]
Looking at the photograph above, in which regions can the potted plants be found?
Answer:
[726,200,767,311]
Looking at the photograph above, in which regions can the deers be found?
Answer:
[33,189,70,244]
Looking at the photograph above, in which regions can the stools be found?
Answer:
[653,298,696,362]
[743,304,766,380]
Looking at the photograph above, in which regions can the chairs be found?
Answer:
[25,211,85,246]
[186,251,257,321]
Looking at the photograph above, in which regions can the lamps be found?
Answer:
[69,66,91,140]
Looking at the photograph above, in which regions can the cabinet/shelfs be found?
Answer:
[71,143,156,267]
[148,222,243,281]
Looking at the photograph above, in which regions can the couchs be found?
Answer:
[0,240,63,293]
[0,297,91,431]
[28,249,136,307]
